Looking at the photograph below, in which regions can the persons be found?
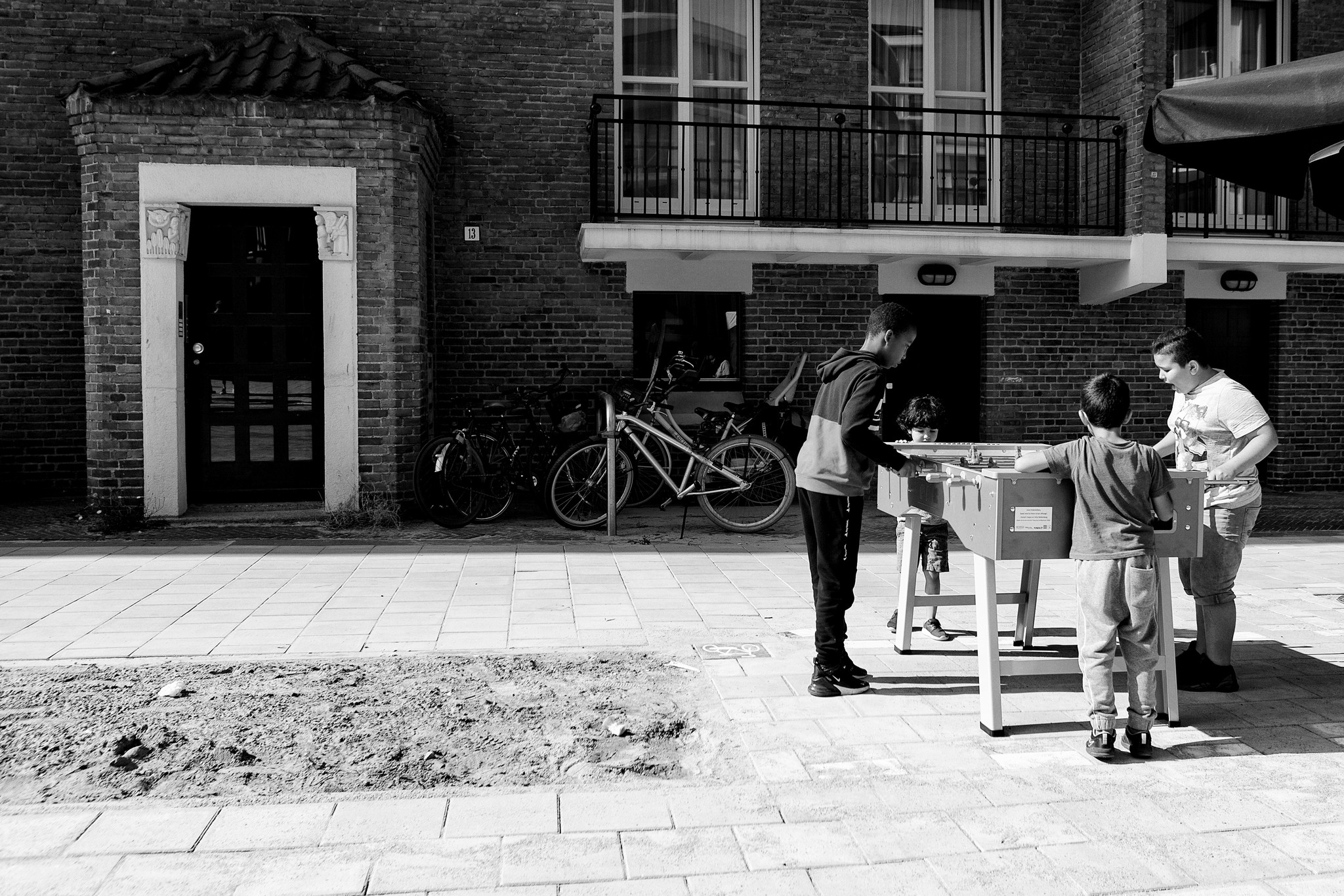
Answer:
[1015,373,1175,759]
[887,394,950,640]
[1150,324,1278,691]
[793,302,921,697]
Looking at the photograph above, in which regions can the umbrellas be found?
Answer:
[1141,51,1344,222]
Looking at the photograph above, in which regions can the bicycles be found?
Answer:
[410,347,798,534]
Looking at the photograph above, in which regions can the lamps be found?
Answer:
[918,264,957,286]
[1220,270,1258,292]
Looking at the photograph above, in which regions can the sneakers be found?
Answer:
[921,618,949,640]
[885,609,898,632]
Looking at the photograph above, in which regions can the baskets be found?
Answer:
[544,386,588,434]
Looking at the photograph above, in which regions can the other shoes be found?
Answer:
[841,651,868,679]
[1085,728,1116,757]
[1121,726,1153,758]
[808,656,869,697]
[1175,640,1239,692]
